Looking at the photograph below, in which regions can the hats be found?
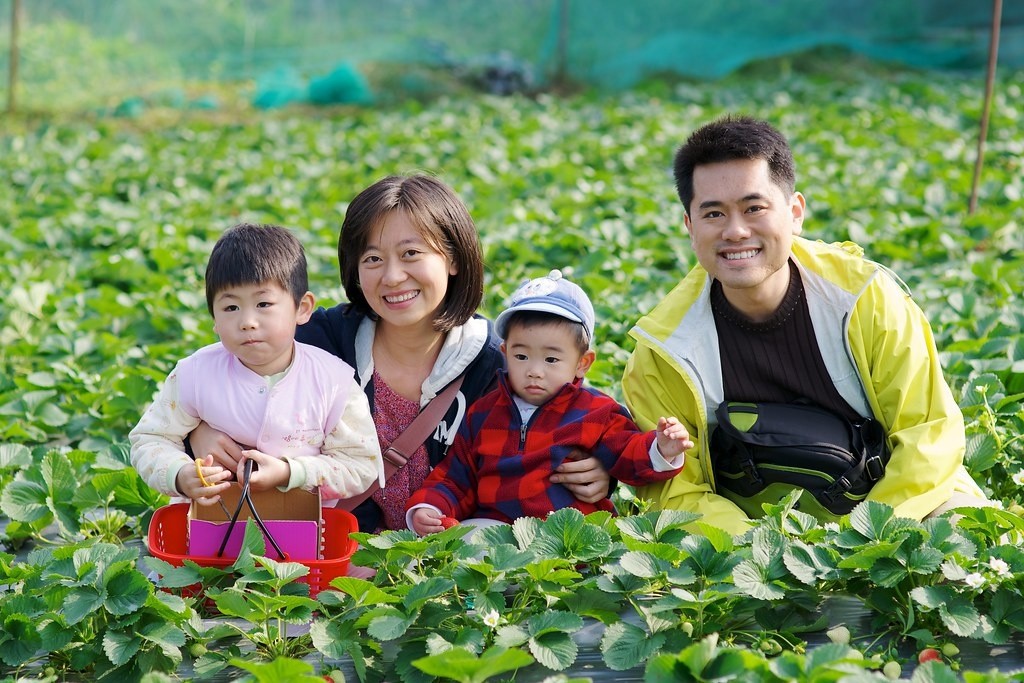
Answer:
[493,269,595,340]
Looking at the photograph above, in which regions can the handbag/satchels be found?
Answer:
[710,400,890,530]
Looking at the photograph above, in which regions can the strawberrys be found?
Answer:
[918,649,941,665]
[436,518,460,530]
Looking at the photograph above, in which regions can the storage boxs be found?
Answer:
[187,480,323,599]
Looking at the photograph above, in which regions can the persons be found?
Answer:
[127,222,385,511]
[620,110,992,544]
[183,168,508,535]
[403,276,695,539]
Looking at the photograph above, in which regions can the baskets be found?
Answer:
[149,458,359,622]
